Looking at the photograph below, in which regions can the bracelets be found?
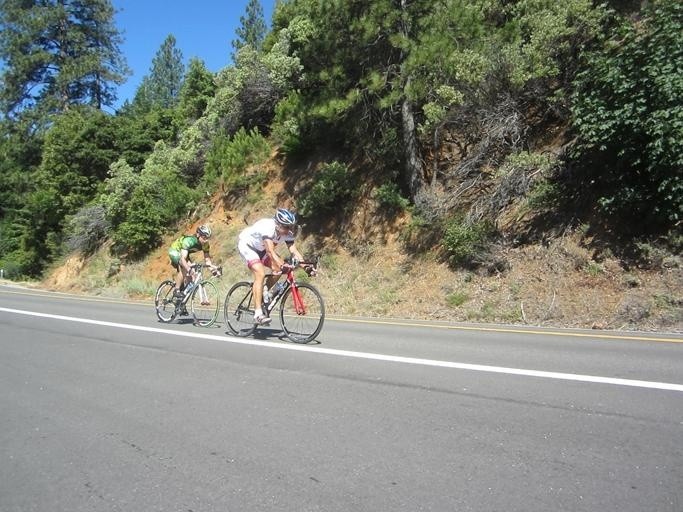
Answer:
[304,265,313,274]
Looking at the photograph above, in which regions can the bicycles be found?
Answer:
[223,250,328,346]
[152,254,225,328]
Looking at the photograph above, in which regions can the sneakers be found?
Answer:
[254,314,271,325]
[173,289,184,297]
[180,306,188,316]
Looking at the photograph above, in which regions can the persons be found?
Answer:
[168,224,221,315]
[237,207,316,325]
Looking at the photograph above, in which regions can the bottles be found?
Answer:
[183,281,196,295]
[262,284,270,304]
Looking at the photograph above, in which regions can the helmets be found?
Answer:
[197,224,212,238]
[275,207,295,226]
